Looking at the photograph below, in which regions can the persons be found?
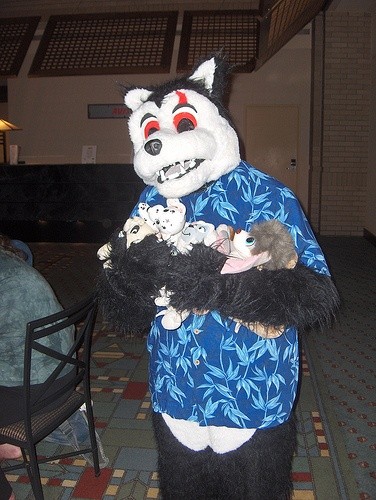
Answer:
[1,247,78,474]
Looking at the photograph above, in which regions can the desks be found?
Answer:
[0,163,148,239]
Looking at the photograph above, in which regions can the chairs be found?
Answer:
[1,291,100,500]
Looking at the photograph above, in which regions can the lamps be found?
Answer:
[0,118,21,131]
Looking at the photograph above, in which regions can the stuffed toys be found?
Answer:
[97,200,299,338]
[97,56,342,499]
